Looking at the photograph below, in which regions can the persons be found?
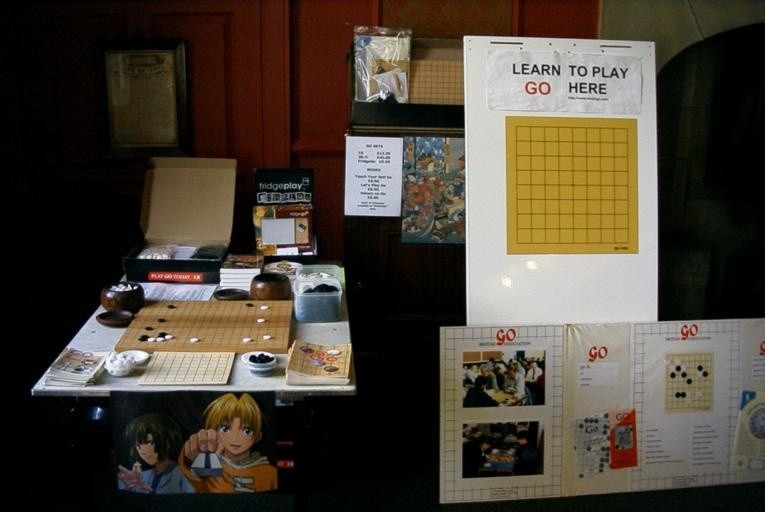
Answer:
[118,392,277,493]
[463,422,544,478]
[460,349,545,407]
[404,134,465,242]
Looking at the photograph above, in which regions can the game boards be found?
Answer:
[115,301,293,354]
[505,116,639,254]
[666,354,713,410]
[137,351,235,385]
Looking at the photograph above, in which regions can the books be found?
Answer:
[43,346,111,387]
[254,168,317,265]
[285,340,352,385]
[220,254,264,296]
[355,36,410,103]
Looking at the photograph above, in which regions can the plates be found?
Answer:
[242,351,278,369]
[119,349,149,364]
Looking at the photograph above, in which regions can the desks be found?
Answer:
[26,237,357,492]
